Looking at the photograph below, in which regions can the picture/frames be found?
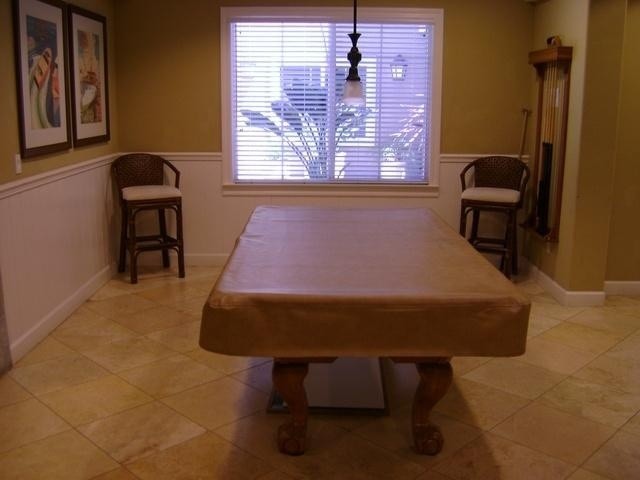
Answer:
[13,0,111,157]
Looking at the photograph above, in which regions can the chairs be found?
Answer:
[110,151,188,284]
[457,155,530,280]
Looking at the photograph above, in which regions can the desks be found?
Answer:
[201,203,536,458]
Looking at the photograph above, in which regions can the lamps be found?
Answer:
[344,0,365,100]
[389,54,408,81]
